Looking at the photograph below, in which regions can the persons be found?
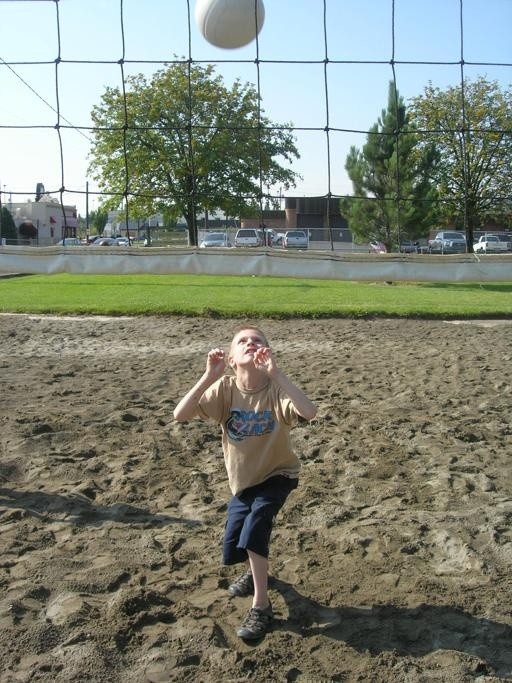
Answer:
[173,325,318,641]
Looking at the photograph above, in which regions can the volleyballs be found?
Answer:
[194,1,265,50]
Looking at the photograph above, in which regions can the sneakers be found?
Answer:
[238,598,272,639]
[230,573,254,595]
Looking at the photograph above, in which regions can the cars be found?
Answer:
[369,230,512,254]
[58,231,150,246]
[200,228,311,247]
